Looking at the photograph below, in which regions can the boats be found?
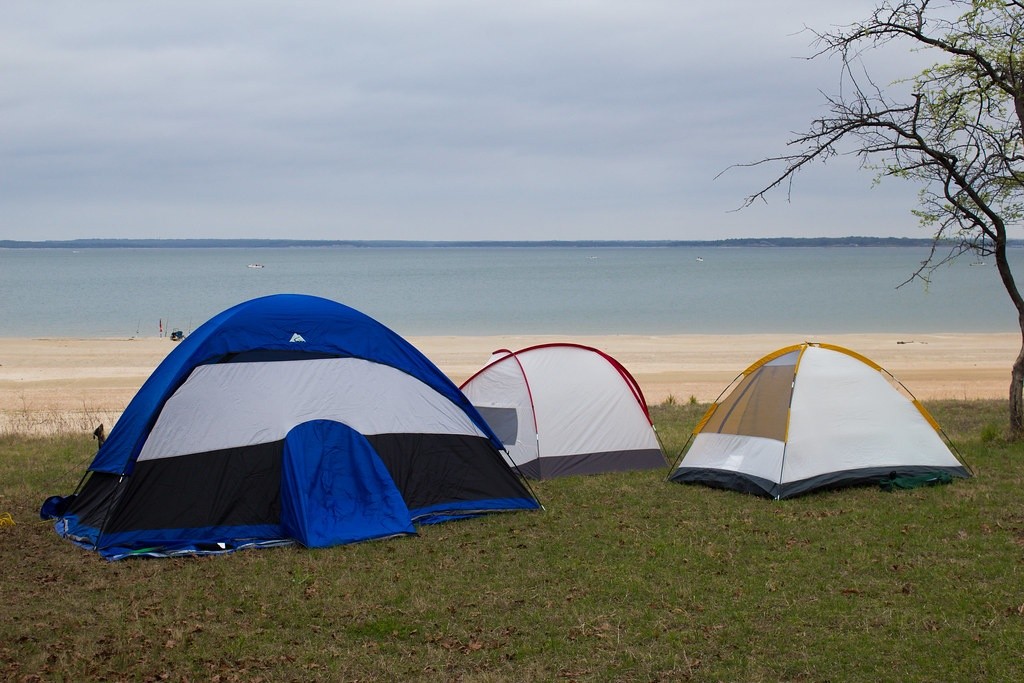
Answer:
[246,264,264,269]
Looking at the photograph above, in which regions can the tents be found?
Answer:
[666,331,976,505]
[41,294,549,563]
[461,342,666,479]
